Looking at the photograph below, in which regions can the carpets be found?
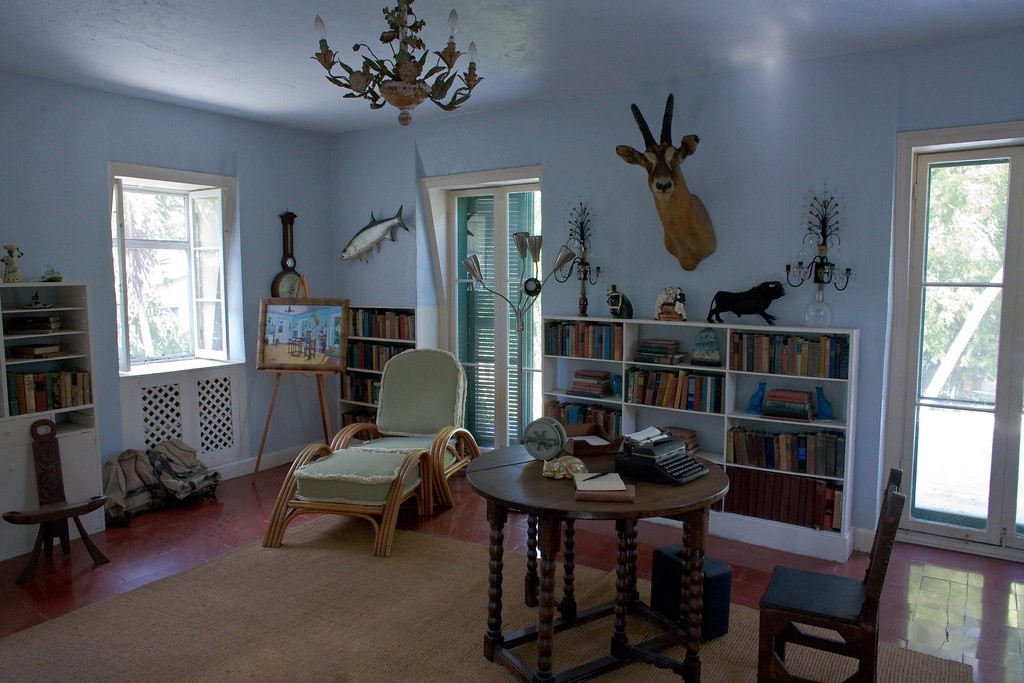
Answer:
[0,514,974,683]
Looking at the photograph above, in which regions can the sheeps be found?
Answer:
[615,91,717,272]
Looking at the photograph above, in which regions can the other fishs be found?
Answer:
[339,204,410,265]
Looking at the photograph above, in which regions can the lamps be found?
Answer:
[461,232,575,512]
[554,192,604,317]
[310,0,485,127]
[785,182,856,327]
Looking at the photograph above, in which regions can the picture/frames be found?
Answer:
[255,297,349,373]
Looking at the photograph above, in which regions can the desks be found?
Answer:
[465,444,729,683]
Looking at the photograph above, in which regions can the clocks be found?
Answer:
[270,212,305,299]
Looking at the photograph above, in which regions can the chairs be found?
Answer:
[330,348,481,512]
[1,419,111,582]
[756,467,906,683]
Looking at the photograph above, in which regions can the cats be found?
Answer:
[542,455,589,479]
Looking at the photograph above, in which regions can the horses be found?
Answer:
[607,285,633,319]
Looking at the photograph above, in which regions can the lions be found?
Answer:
[706,281,785,327]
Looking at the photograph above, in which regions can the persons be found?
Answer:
[0,245,25,283]
[654,286,687,322]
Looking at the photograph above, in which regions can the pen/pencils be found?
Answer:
[582,472,610,482]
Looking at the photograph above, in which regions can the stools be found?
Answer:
[262,443,434,558]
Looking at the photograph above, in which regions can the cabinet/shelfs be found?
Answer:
[0,279,106,562]
[542,316,860,563]
[337,305,416,447]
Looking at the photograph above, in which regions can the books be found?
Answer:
[730,332,849,379]
[544,399,620,436]
[342,410,381,441]
[726,425,845,479]
[348,310,414,340]
[7,369,91,416]
[544,321,623,360]
[710,465,843,534]
[340,372,381,404]
[566,369,613,399]
[6,345,67,359]
[573,471,636,503]
[635,339,687,364]
[761,389,815,422]
[346,342,415,372]
[663,426,700,454]
[3,315,61,334]
[624,366,725,413]
